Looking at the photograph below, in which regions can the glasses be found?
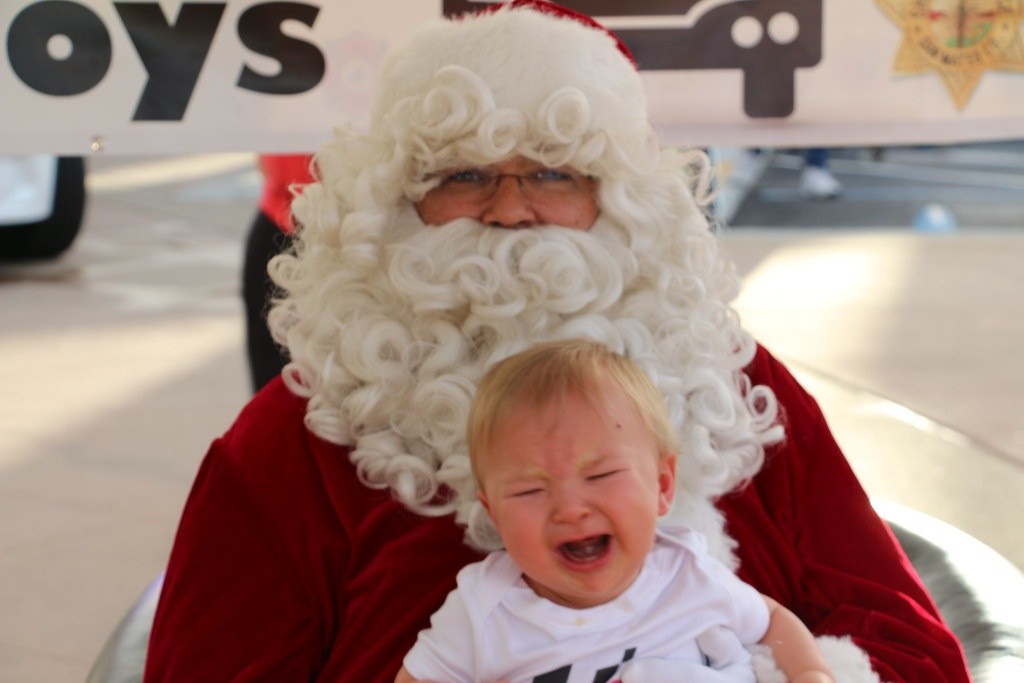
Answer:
[431,167,583,212]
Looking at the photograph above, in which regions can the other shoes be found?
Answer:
[798,163,840,199]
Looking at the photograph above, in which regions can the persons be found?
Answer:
[138,0,973,683]
[798,149,844,200]
[388,341,835,683]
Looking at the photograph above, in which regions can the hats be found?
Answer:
[368,0,641,150]
